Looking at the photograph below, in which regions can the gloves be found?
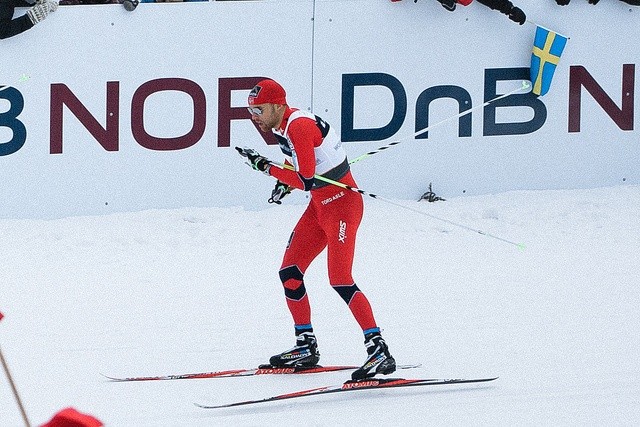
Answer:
[271,180,291,205]
[509,7,526,24]
[236,147,270,171]
[26,1,59,25]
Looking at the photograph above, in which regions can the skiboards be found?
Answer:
[98,364,500,408]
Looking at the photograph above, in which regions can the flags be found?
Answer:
[530,26,570,94]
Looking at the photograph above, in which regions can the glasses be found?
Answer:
[246,105,271,115]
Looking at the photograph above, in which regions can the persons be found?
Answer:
[236,77,396,379]
[1,0,58,39]
[476,0,527,27]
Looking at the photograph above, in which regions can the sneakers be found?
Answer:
[352,352,396,380]
[270,344,320,368]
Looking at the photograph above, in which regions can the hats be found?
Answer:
[248,79,286,106]
